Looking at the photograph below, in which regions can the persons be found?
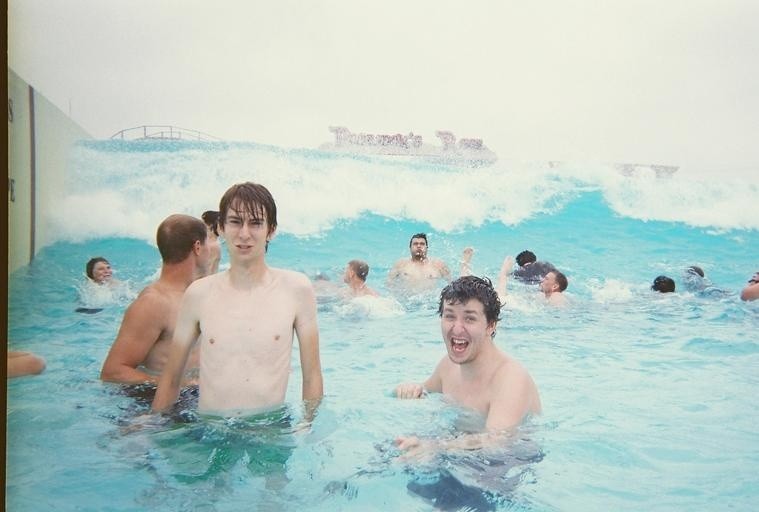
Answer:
[312,269,340,294]
[75,255,129,315]
[386,231,474,292]
[495,254,580,309]
[680,263,737,305]
[200,208,223,277]
[739,268,759,302]
[391,274,547,511]
[647,274,680,296]
[507,248,560,287]
[118,178,325,494]
[97,212,214,438]
[335,256,385,301]
[7,347,47,382]
